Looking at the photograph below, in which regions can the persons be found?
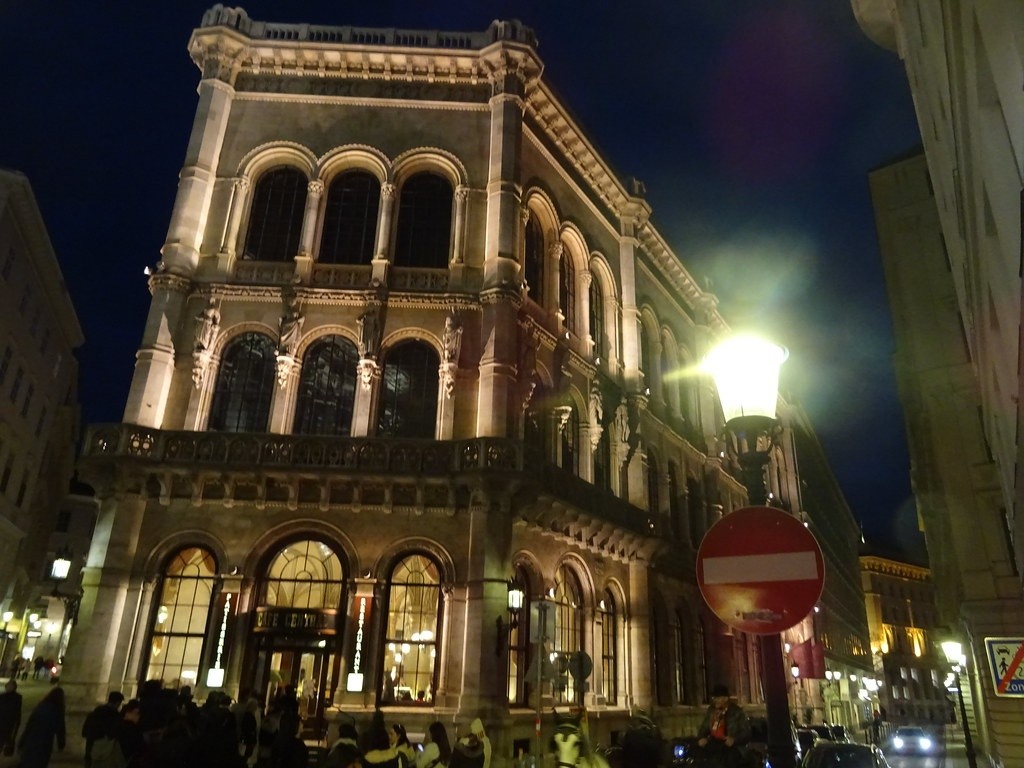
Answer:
[9,652,53,681]
[18,688,65,768]
[314,671,493,768]
[81,670,319,768]
[693,683,753,767]
[0,681,21,757]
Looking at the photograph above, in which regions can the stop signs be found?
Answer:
[696,505,826,637]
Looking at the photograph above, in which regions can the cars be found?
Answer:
[891,726,931,758]
[800,738,891,768]
[603,707,860,768]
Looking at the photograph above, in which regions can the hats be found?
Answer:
[712,683,730,697]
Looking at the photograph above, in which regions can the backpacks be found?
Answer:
[90,726,133,768]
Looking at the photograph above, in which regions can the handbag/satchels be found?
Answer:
[238,739,246,757]
[4,726,18,757]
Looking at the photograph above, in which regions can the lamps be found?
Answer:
[494,576,526,658]
[51,548,80,627]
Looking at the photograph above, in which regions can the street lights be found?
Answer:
[937,631,977,768]
[699,329,790,768]
